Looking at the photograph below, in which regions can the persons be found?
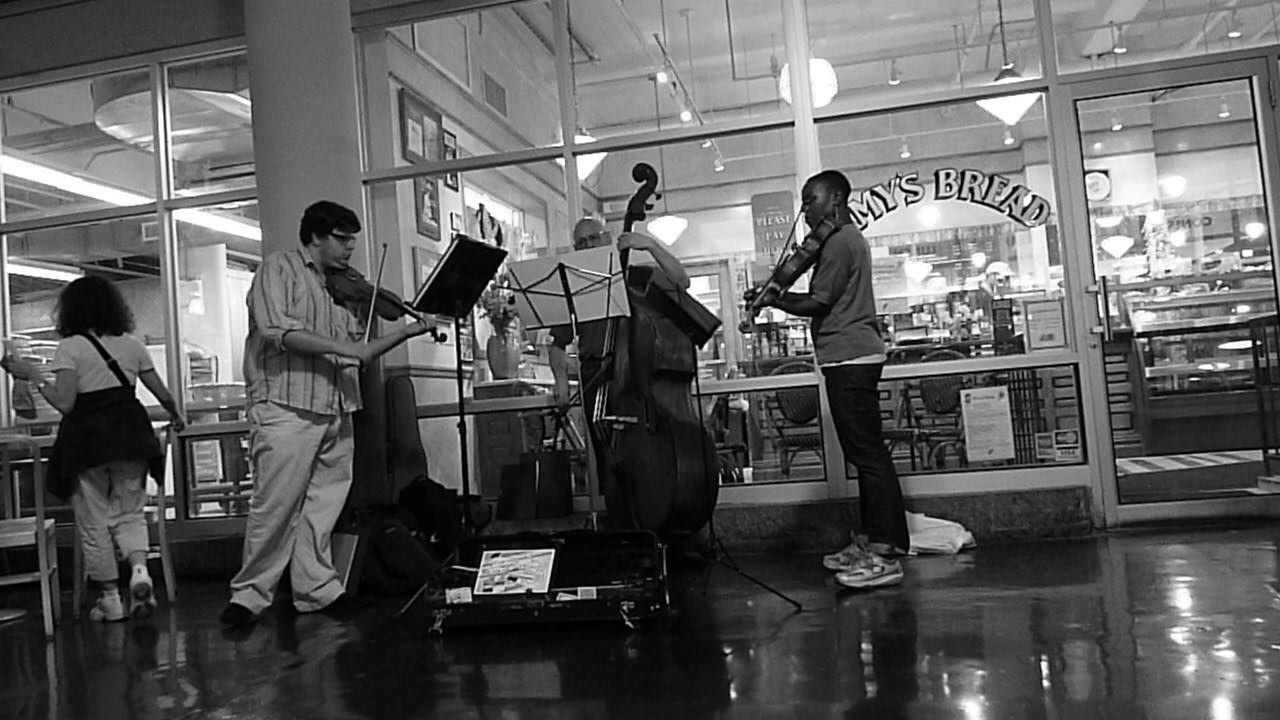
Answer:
[742,170,910,589]
[550,216,703,662]
[0,276,185,622]
[221,199,438,628]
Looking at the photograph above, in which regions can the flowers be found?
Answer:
[476,288,519,349]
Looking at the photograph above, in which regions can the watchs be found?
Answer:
[37,380,50,392]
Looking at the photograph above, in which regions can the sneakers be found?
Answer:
[823,530,870,570]
[834,548,903,589]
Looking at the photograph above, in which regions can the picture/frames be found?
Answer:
[396,83,461,243]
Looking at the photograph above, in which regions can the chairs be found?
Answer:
[888,350,981,467]
[0,424,174,640]
[755,361,829,479]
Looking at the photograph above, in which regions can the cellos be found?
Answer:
[592,161,719,608]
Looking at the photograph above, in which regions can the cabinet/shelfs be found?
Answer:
[1130,281,1275,395]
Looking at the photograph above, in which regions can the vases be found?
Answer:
[486,334,522,379]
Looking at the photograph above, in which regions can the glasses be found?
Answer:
[330,233,356,246]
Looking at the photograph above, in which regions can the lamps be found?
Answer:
[897,139,912,159]
[1109,25,1133,61]
[1109,115,1125,132]
[1000,126,1015,145]
[974,0,1044,130]
[1216,96,1233,120]
[1223,10,1243,42]
[551,0,609,187]
[884,57,905,90]
[644,30,737,248]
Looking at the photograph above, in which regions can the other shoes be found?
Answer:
[129,574,157,620]
[89,595,125,621]
[324,592,368,618]
[220,603,250,627]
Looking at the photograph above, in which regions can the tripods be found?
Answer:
[645,281,802,615]
[399,232,509,620]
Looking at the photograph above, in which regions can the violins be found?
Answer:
[328,261,450,344]
[737,211,844,334]
